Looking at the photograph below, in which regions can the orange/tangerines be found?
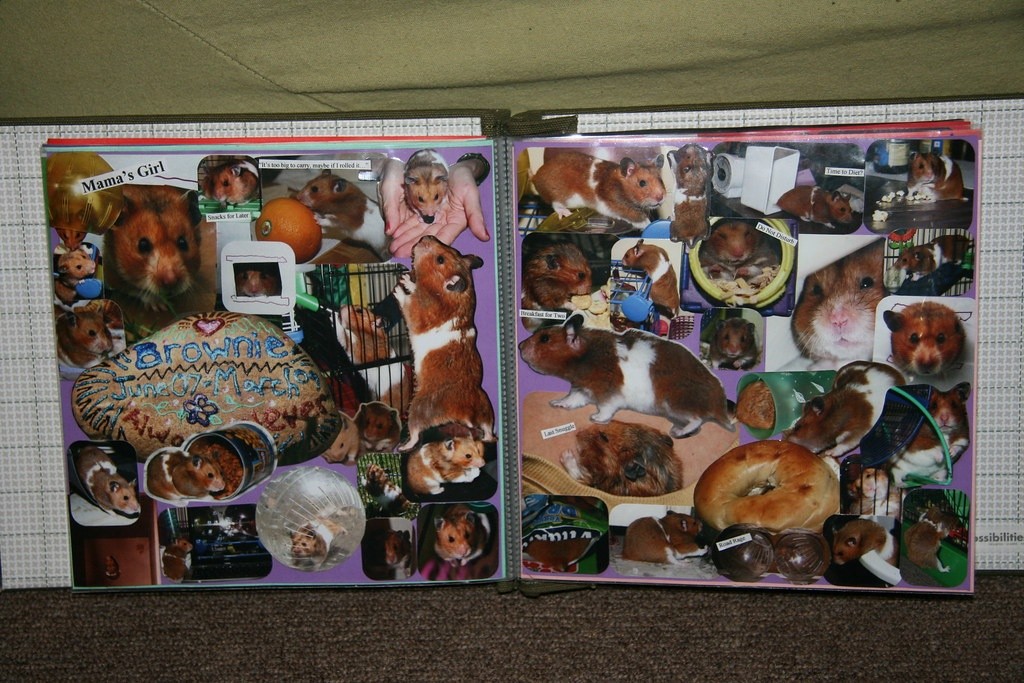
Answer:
[256,198,322,264]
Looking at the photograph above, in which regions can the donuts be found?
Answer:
[692,439,841,537]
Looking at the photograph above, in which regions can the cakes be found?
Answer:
[70,311,340,467]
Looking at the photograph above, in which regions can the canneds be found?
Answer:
[873,140,951,175]
[181,422,278,501]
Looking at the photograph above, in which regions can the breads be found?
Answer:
[570,294,607,315]
[736,381,773,430]
